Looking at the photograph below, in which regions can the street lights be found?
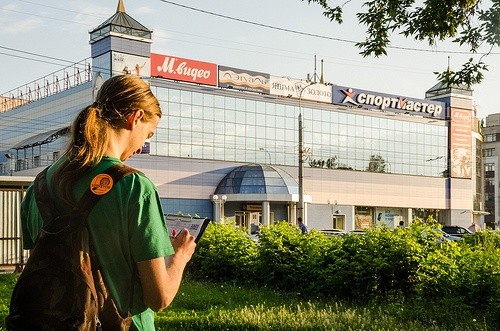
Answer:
[298,82,318,228]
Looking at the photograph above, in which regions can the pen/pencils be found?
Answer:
[172,229,177,238]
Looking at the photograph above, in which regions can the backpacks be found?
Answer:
[5,163,144,331]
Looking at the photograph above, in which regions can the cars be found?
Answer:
[422,229,461,245]
[443,225,473,237]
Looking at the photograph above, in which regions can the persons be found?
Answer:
[19,74,196,331]
[297,217,307,235]
[91,72,104,103]
